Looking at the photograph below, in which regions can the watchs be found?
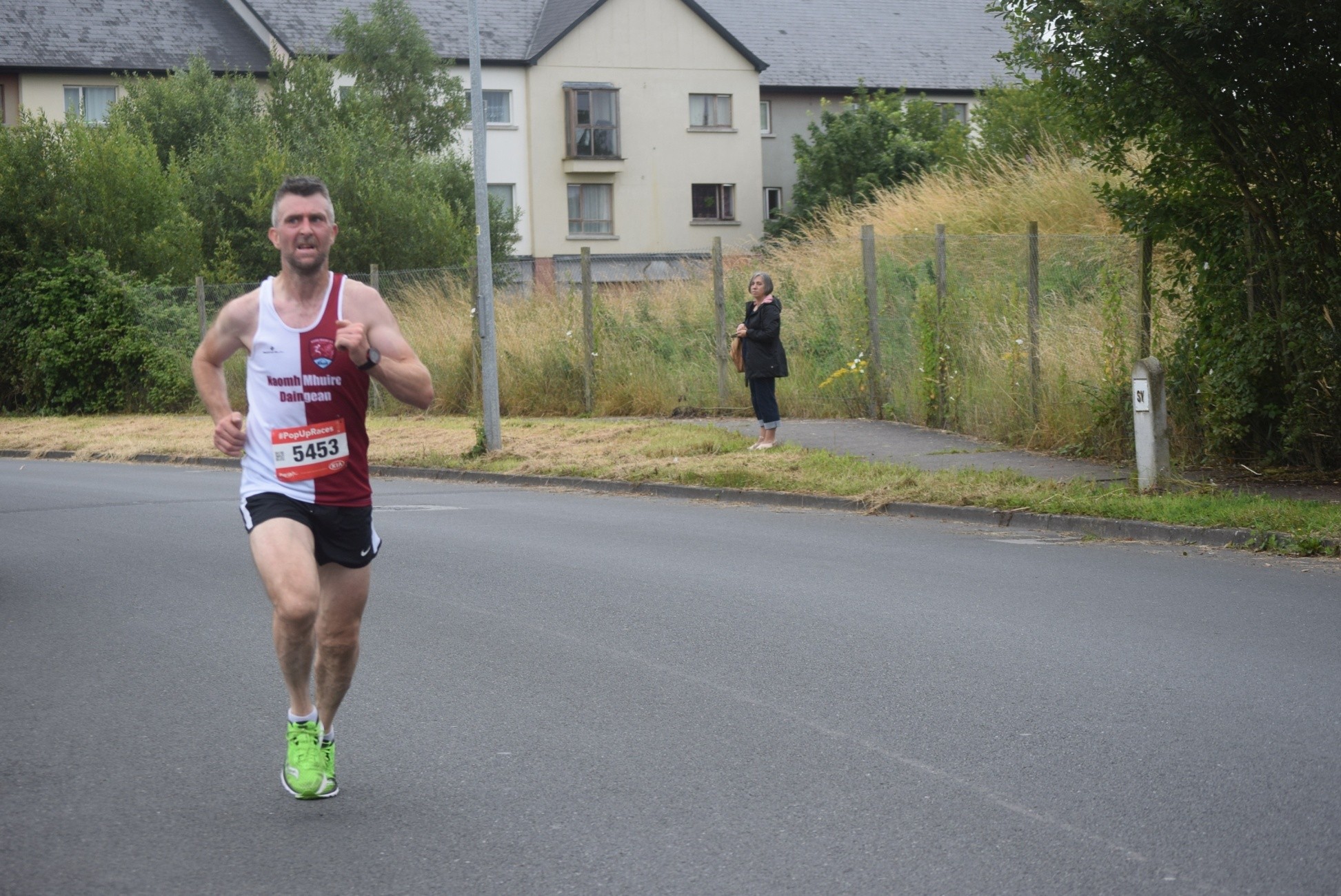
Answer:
[359,348,382,370]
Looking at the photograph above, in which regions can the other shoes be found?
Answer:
[748,437,766,449]
[749,441,776,449]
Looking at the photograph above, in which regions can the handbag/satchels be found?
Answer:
[730,338,744,373]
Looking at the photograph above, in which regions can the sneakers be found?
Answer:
[296,738,339,797]
[281,723,325,797]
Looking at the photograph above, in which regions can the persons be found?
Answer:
[190,177,433,800]
[735,272,789,451]
